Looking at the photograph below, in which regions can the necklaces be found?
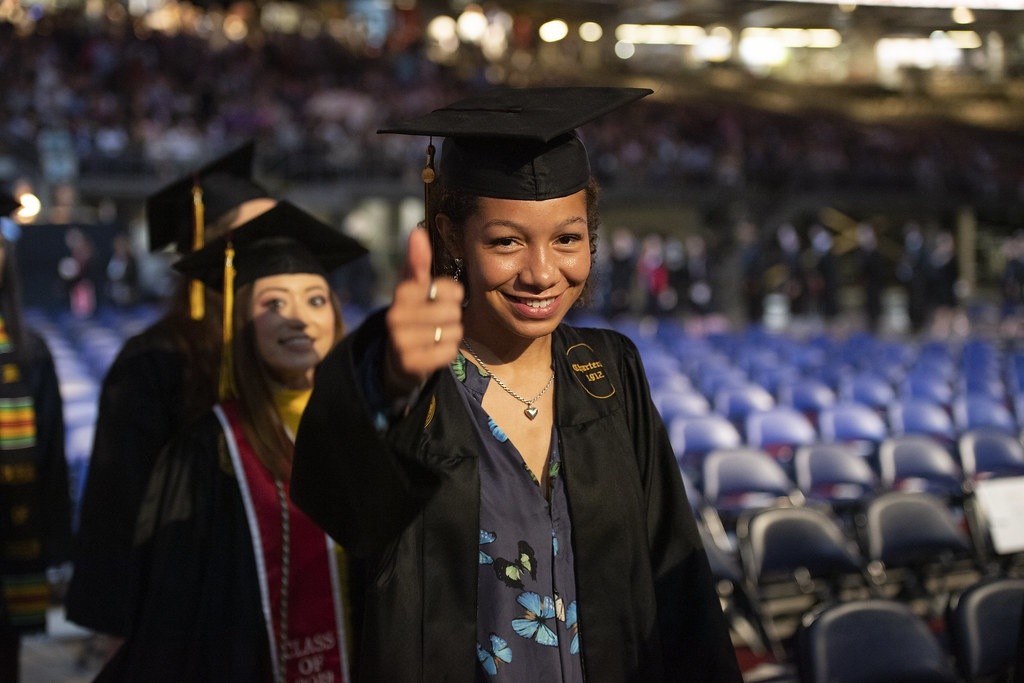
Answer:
[460,335,554,420]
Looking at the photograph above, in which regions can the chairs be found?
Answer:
[25,301,1024,683]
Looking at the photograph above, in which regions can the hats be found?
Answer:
[171,200,368,402]
[146,139,275,319]
[376,86,654,280]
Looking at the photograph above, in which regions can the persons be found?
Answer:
[289,87,743,683]
[0,182,78,683]
[0,169,1024,329]
[65,142,281,632]
[94,199,373,683]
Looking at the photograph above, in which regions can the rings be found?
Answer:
[435,326,441,341]
[430,283,437,299]
